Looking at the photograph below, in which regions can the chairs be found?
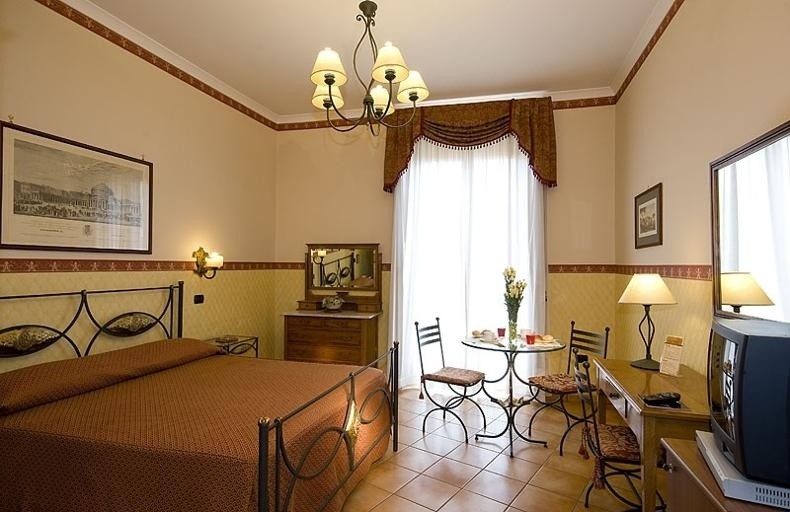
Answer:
[527,320,609,456]
[414,317,486,444]
[573,346,669,512]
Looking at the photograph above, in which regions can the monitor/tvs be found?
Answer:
[707,310,790,490]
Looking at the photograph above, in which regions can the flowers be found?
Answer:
[503,268,528,323]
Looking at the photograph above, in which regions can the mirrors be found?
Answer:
[304,241,381,289]
[709,118,789,330]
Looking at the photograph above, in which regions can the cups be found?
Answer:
[526,331,536,345]
[520,329,532,338]
[484,332,494,340]
[497,326,506,337]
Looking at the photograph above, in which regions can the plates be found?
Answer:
[536,340,552,343]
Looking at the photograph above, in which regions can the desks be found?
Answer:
[659,437,790,512]
[461,329,567,458]
[586,354,718,512]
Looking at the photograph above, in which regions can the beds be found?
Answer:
[1,277,402,512]
[320,253,374,288]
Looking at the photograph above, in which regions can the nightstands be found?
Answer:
[202,334,258,358]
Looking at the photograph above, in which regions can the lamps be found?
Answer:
[617,272,679,372]
[720,273,778,312]
[310,0,430,137]
[191,247,225,280]
[313,251,326,265]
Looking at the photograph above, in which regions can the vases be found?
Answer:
[508,320,521,343]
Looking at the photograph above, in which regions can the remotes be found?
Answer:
[642,392,681,406]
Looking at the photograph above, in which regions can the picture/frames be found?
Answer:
[0,121,157,250]
[634,181,664,247]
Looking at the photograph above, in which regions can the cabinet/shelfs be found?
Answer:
[283,289,383,367]
